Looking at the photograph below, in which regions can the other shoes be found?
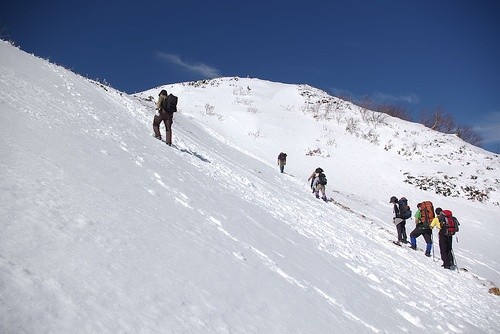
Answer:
[393,241,401,246]
[402,239,407,244]
[425,250,430,257]
[166,143,171,146]
[156,135,161,140]
[409,246,416,250]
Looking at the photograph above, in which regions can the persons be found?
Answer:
[389,196,407,246]
[408,203,432,257]
[308,167,326,201]
[153,89,174,147]
[429,207,454,270]
[278,152,286,173]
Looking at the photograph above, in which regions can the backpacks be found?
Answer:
[419,201,435,227]
[438,210,460,237]
[395,197,412,219]
[316,168,324,173]
[318,173,328,186]
[161,94,178,113]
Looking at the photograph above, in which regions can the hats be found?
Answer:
[390,196,398,203]
[158,90,167,96]
